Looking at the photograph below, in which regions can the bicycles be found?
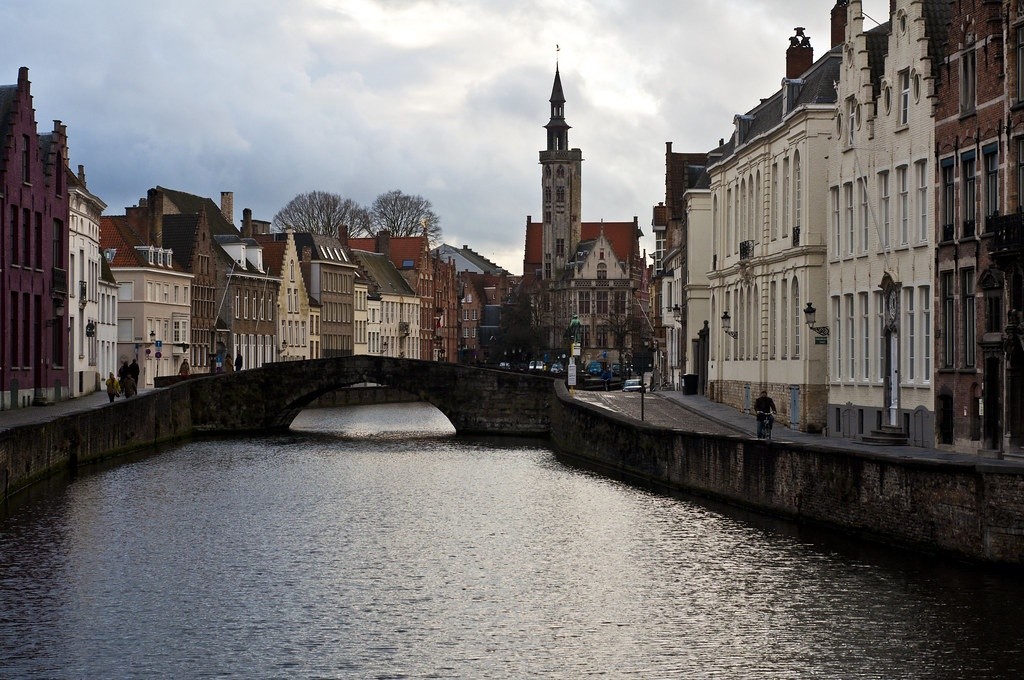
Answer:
[756,411,774,440]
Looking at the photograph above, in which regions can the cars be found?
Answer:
[499,359,510,368]
[529,361,546,372]
[608,363,624,374]
[621,379,647,393]
[551,364,563,374]
[588,363,603,376]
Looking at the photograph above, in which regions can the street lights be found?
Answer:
[504,351,507,372]
[553,311,556,349]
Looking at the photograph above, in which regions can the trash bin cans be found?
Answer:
[680,373,700,395]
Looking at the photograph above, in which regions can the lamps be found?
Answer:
[721,311,738,339]
[803,302,830,335]
[279,339,287,354]
[143,331,155,348]
[45,302,64,327]
[380,342,388,354]
[673,304,686,327]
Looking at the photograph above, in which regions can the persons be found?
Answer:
[601,367,611,391]
[105,358,140,404]
[754,390,777,438]
[225,353,243,373]
[178,359,189,377]
